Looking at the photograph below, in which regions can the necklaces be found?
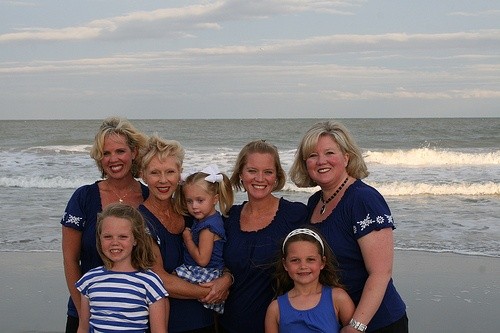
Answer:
[103,179,136,204]
[319,177,349,215]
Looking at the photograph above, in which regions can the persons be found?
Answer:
[74,202,170,333]
[60,118,150,333]
[221,138,307,333]
[136,132,235,333]
[289,122,408,333]
[165,172,234,314]
[264,225,356,333]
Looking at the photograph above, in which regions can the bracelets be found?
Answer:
[349,317,368,332]
